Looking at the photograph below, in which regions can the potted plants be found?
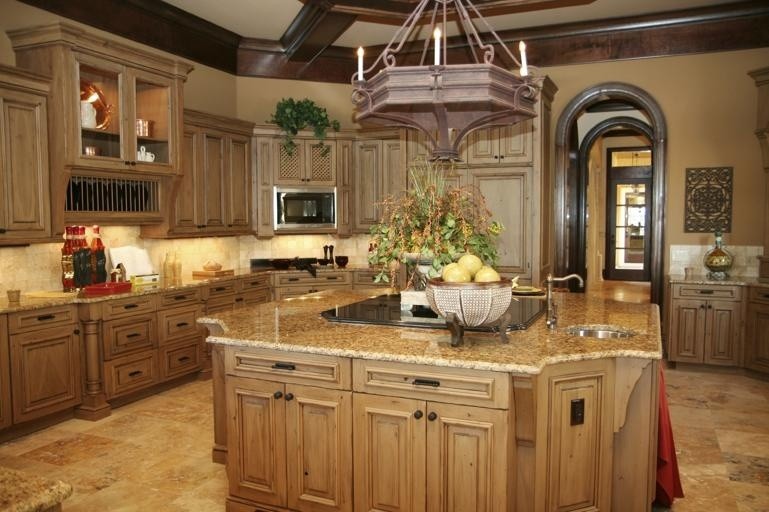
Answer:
[366,156,505,291]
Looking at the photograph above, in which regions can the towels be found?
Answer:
[656,368,685,509]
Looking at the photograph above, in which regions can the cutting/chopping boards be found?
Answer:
[192,270,233,276]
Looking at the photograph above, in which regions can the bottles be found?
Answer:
[79,226,92,285]
[73,226,81,287]
[91,225,107,283]
[164,248,172,277]
[173,248,182,276]
[62,227,76,292]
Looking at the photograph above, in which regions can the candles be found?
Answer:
[431,26,443,65]
[517,37,529,76]
[354,44,368,82]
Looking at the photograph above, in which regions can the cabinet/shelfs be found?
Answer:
[157,290,201,378]
[2,89,53,241]
[748,289,763,372]
[8,300,79,424]
[537,362,613,509]
[0,319,15,430]
[204,287,230,379]
[61,53,178,166]
[354,141,397,224]
[100,300,161,394]
[224,348,351,510]
[412,113,531,160]
[182,128,249,231]
[257,135,337,187]
[234,280,273,305]
[353,359,507,511]
[671,284,745,373]
[274,274,352,300]
[408,169,530,275]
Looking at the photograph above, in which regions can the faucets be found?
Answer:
[542,269,586,323]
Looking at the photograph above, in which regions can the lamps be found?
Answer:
[341,1,533,161]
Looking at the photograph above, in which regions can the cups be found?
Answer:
[7,290,21,302]
[137,146,144,160]
[136,118,151,136]
[144,152,155,161]
[81,104,97,129]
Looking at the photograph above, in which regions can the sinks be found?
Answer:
[569,319,634,341]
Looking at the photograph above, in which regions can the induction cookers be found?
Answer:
[251,258,317,273]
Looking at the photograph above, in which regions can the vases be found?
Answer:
[703,226,734,282]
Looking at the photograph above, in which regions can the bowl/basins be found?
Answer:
[203,265,222,271]
[318,259,328,264]
[271,260,290,269]
[335,256,348,269]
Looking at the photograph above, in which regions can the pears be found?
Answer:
[458,253,483,277]
[442,263,470,283]
[475,264,502,281]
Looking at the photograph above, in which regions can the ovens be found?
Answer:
[273,185,337,229]
